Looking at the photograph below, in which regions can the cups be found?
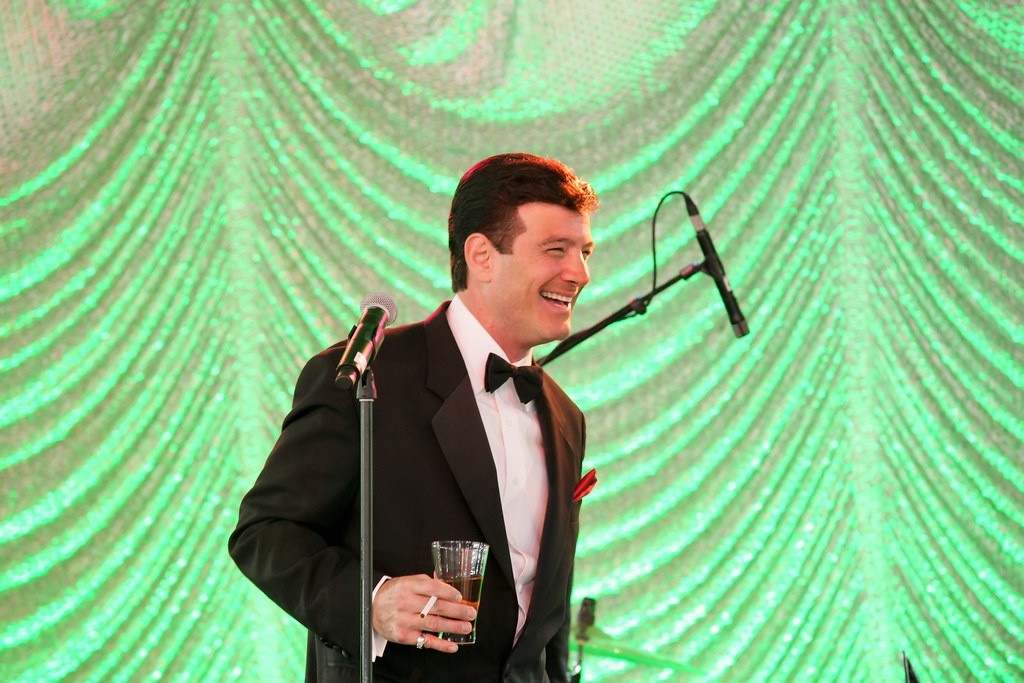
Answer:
[430,540,489,644]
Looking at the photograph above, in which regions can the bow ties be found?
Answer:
[484,353,543,405]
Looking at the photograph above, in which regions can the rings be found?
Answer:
[417,633,425,649]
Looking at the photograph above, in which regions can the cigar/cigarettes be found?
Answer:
[419,596,438,619]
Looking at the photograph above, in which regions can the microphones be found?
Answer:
[684,196,751,339]
[334,293,399,389]
[577,597,597,631]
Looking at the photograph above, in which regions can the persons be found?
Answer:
[227,152,600,683]
